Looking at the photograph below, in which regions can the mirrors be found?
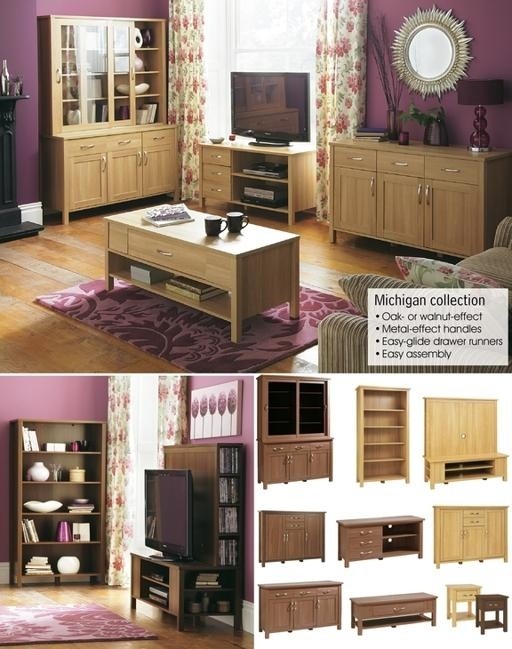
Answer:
[389,4,476,102]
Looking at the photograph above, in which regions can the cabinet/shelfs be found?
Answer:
[36,15,183,137]
[355,385,412,487]
[9,420,108,587]
[259,580,344,639]
[163,442,247,568]
[262,510,286,567]
[197,138,317,226]
[64,134,143,211]
[283,510,305,565]
[423,160,482,254]
[433,504,508,568]
[381,516,426,560]
[423,396,498,458]
[255,374,331,440]
[302,511,327,563]
[329,142,376,240]
[141,130,176,198]
[336,516,382,567]
[130,552,246,638]
[257,439,335,489]
[376,150,425,251]
[0,94,44,244]
[426,454,509,490]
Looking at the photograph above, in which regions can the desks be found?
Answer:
[103,203,302,346]
[350,592,438,636]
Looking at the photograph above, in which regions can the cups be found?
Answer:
[226,212,249,234]
[204,217,228,237]
[399,132,409,145]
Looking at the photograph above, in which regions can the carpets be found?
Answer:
[33,278,365,372]
[0,601,159,647]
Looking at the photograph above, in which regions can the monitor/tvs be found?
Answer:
[231,72,311,147]
[145,469,195,563]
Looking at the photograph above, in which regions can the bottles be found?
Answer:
[1,57,10,97]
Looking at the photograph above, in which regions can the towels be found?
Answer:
[141,203,195,228]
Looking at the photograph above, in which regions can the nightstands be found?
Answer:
[445,584,482,626]
[474,594,510,636]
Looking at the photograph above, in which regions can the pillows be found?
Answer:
[393,254,512,297]
[338,272,439,321]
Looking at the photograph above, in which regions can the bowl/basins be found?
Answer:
[210,138,224,144]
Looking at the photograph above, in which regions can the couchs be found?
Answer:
[316,217,512,374]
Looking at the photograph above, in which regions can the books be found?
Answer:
[195,572,222,588]
[171,278,216,294]
[21,425,40,451]
[354,132,388,136]
[21,519,40,543]
[353,139,381,142]
[358,128,385,132]
[218,507,238,533]
[136,102,158,124]
[165,280,227,301]
[144,217,194,228]
[218,540,236,566]
[219,447,241,473]
[355,135,382,140]
[218,478,240,503]
[67,504,95,514]
[25,556,53,575]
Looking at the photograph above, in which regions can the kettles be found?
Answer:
[424,121,447,146]
[57,521,72,542]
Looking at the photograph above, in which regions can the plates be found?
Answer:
[24,499,64,514]
